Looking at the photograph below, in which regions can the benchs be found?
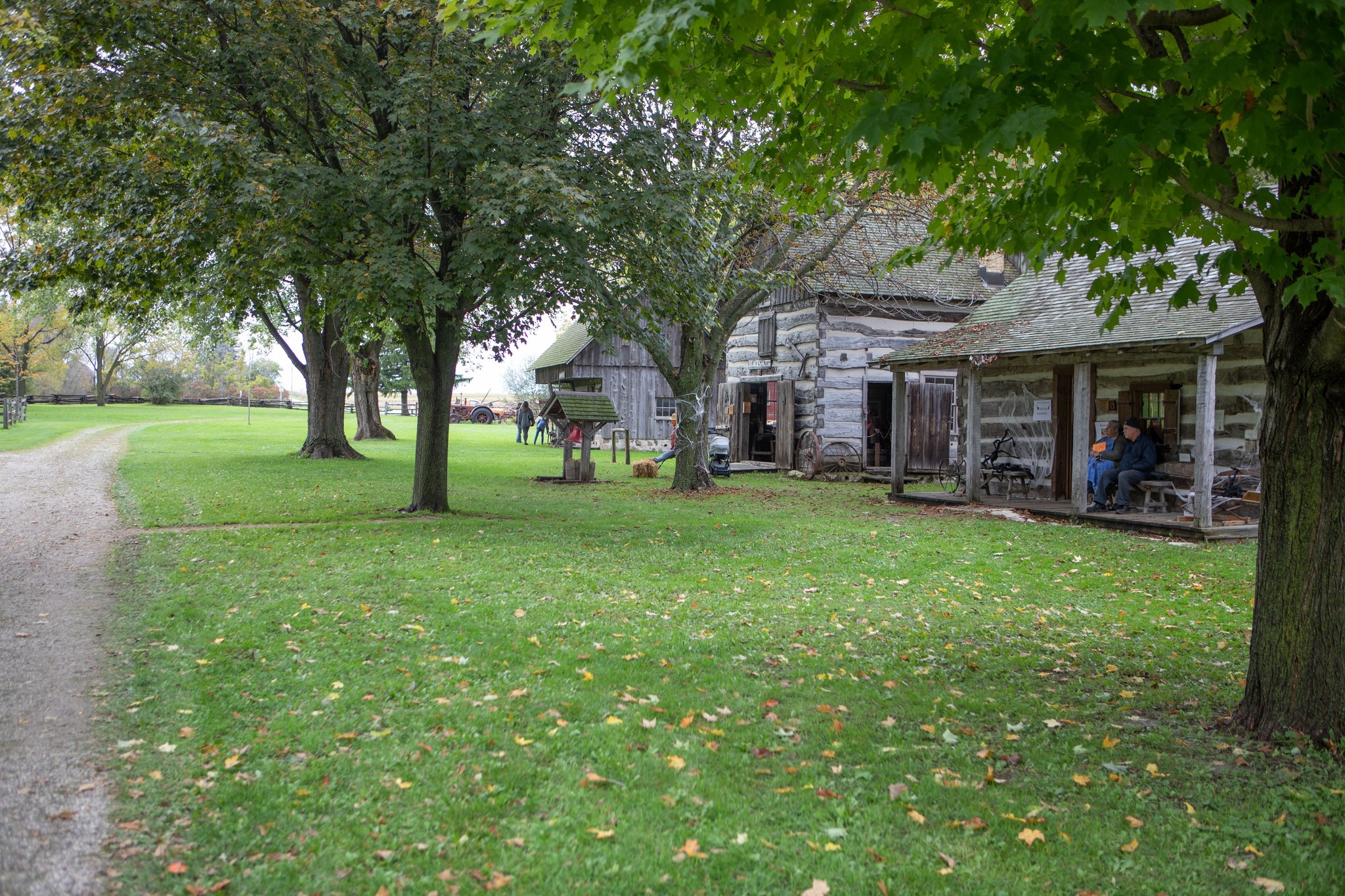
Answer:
[1138,480,1173,514]
[980,469,1027,500]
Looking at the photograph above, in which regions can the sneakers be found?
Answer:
[1086,501,1107,513]
[1116,506,1130,514]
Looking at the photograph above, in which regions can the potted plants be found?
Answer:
[565,456,595,481]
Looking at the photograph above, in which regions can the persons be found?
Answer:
[514,401,550,445]
[1084,418,1157,514]
[653,414,677,462]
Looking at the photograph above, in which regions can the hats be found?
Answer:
[1124,416,1142,429]
[670,413,677,420]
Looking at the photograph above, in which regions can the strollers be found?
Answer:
[707,432,731,478]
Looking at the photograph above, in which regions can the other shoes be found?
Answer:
[523,442,528,445]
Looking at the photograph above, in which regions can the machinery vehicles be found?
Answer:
[448,399,504,425]
